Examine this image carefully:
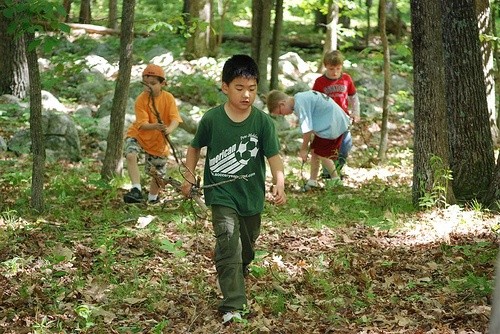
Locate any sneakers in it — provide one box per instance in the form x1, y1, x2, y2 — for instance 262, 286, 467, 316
222, 312, 241, 325
123, 187, 145, 203
147, 196, 160, 204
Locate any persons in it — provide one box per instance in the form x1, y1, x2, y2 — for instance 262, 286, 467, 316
123, 65, 182, 204
267, 90, 352, 191
182, 54, 286, 326
312, 50, 361, 180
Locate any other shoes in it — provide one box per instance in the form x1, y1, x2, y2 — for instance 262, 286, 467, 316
305, 179, 327, 192
331, 177, 341, 184
320, 173, 342, 180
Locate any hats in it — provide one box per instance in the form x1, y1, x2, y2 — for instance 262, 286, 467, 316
142, 65, 166, 80
